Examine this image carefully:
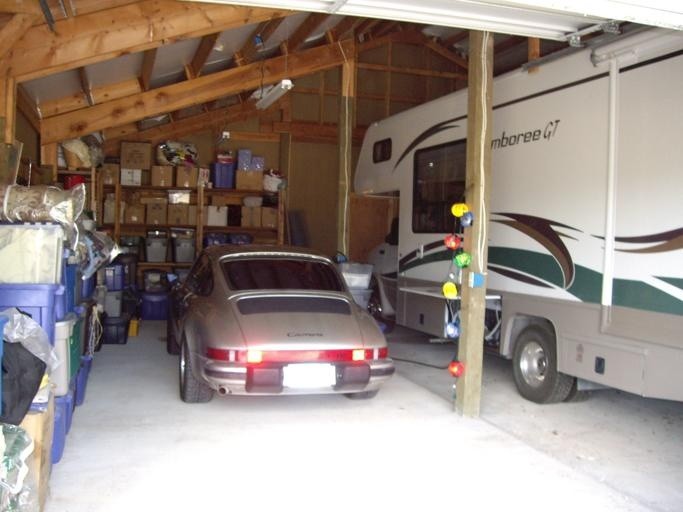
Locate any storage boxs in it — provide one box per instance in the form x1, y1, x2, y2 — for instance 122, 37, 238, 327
0, 249, 93, 511
94, 140, 277, 345
342, 262, 374, 308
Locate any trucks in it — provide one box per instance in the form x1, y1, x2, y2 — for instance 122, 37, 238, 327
352, 25, 683, 405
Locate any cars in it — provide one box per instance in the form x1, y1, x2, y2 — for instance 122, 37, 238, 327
167, 245, 395, 404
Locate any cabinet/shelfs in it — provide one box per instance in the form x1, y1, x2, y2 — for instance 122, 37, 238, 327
58, 164, 285, 269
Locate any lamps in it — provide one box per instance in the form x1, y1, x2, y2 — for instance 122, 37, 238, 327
255, 18, 293, 112
